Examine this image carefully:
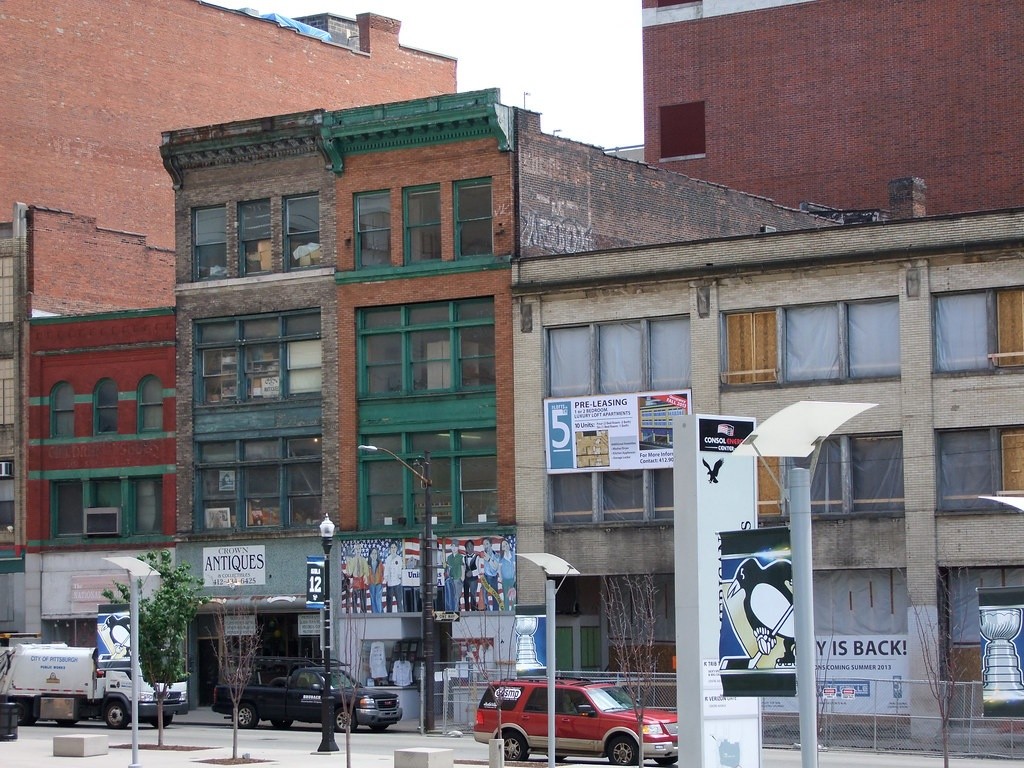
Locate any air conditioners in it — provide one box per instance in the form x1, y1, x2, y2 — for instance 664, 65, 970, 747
0, 462, 14, 478
82, 506, 123, 536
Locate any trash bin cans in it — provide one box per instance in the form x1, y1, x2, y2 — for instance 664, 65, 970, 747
0, 703, 22, 739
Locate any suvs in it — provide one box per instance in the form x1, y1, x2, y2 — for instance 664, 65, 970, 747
473, 676, 681, 766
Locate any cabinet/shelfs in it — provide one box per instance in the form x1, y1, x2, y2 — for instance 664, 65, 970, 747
221, 349, 279, 400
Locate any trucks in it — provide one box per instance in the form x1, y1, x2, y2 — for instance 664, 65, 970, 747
1, 639, 189, 728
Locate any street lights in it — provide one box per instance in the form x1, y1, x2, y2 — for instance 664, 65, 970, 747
356, 443, 450, 734
317, 512, 347, 752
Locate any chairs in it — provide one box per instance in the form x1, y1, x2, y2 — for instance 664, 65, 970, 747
560, 694, 577, 714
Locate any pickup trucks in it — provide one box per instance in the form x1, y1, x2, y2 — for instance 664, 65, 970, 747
211, 667, 403, 732
